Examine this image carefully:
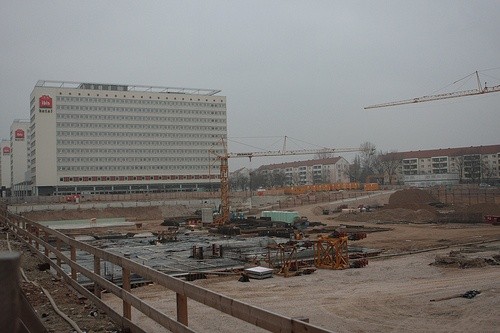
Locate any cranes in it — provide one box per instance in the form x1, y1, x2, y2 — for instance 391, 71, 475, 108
209, 135, 377, 227
364, 65, 500, 110
